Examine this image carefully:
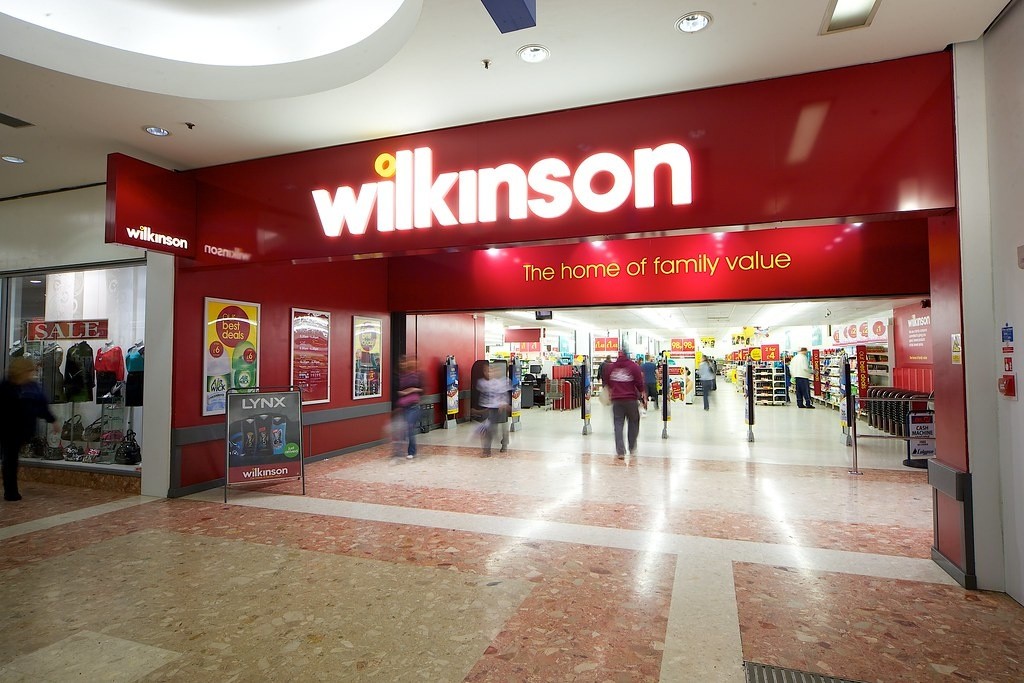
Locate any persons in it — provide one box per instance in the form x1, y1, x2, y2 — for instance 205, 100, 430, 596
597, 353, 659, 409
478, 363, 520, 458
10, 340, 24, 358
0, 358, 60, 501
398, 356, 423, 459
602, 349, 647, 460
699, 356, 717, 410
125, 341, 144, 406
790, 348, 816, 408
785, 358, 791, 403
64, 341, 96, 402
36, 341, 64, 398
94, 341, 124, 404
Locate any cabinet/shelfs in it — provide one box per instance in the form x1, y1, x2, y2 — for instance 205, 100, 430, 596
590, 355, 619, 397
722, 346, 890, 409
